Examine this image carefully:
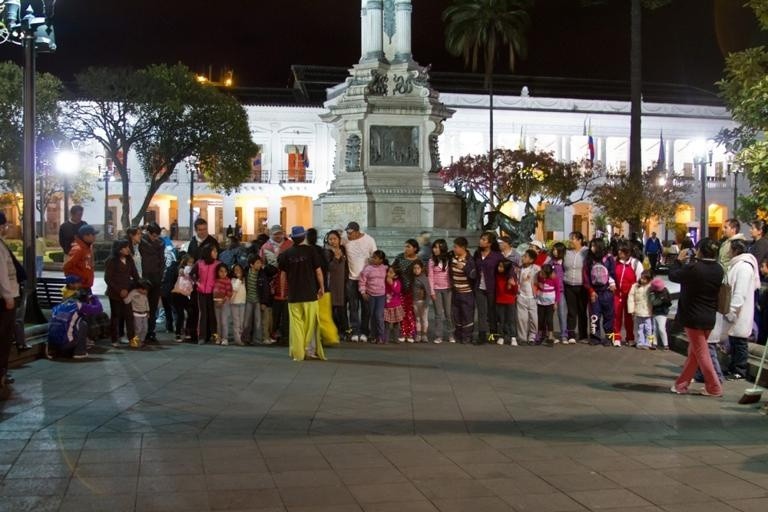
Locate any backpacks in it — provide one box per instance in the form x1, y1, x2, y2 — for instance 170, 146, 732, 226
590, 252, 611, 285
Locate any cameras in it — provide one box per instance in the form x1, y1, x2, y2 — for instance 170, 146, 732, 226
688, 249, 696, 256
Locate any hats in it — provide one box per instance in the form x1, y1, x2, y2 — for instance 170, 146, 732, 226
344, 222, 359, 231
269, 225, 283, 235
78, 224, 100, 237
63, 275, 82, 283
288, 226, 309, 238
651, 279, 665, 292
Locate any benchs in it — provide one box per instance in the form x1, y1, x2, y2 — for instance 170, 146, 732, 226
32, 277, 67, 309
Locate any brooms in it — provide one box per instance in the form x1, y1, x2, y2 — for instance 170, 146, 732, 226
738, 338, 768, 404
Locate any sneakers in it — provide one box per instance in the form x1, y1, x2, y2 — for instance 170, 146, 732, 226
726, 372, 746, 382
670, 384, 680, 394
44, 342, 53, 360
120, 339, 129, 343
86, 336, 95, 350
73, 352, 89, 359
111, 342, 120, 347
173, 329, 282, 345
699, 386, 721, 396
351, 334, 669, 350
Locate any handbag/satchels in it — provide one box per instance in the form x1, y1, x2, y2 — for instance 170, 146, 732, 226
717, 283, 732, 313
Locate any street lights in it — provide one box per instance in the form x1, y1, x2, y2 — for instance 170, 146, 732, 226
56, 149, 82, 222
726, 152, 744, 221
187, 152, 200, 241
192, 64, 237, 92
95, 154, 117, 240
691, 136, 719, 240
0, 0, 58, 349
658, 175, 669, 247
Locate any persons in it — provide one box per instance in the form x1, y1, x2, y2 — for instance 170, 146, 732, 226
665, 239, 725, 400
720, 237, 762, 381
690, 311, 725, 385
10, 317, 33, 354
1, 209, 29, 385
44, 204, 768, 363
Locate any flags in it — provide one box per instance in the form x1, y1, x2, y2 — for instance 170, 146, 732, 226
585, 126, 595, 172
658, 133, 666, 170
